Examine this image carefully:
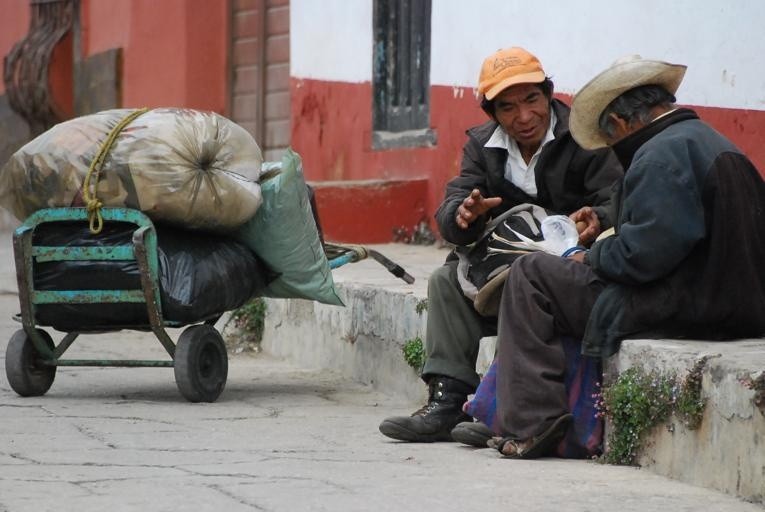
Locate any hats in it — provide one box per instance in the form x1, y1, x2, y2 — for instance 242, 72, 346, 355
566, 54, 690, 155
476, 47, 548, 103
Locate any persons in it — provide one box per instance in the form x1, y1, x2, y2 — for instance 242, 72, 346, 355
488, 54, 764, 460
380, 47, 624, 447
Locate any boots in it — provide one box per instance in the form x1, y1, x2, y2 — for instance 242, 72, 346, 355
448, 416, 496, 449
376, 376, 472, 442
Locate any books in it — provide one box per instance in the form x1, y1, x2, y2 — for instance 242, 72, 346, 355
489, 213, 615, 259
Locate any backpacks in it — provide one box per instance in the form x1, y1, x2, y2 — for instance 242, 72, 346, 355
457, 203, 560, 318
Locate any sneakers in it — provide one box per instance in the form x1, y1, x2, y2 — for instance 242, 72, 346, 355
486, 410, 574, 460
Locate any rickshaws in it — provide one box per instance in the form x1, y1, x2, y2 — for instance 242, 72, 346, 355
4, 205, 416, 402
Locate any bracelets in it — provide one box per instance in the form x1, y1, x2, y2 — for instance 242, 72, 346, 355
561, 246, 586, 256
